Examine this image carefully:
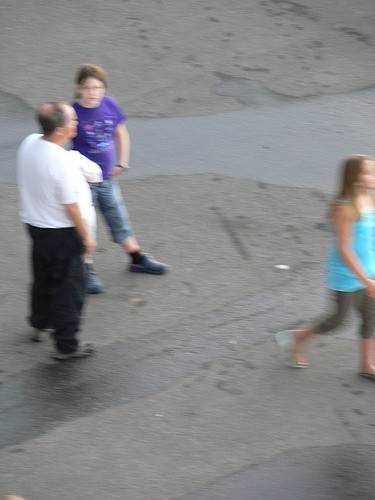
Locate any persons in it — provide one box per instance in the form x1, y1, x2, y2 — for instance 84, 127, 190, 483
16, 102, 98, 360
275, 155, 375, 379
64, 63, 169, 294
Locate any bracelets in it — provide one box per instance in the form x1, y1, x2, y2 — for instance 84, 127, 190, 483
118, 164, 130, 172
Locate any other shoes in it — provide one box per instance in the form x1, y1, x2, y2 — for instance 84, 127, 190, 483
52, 342, 94, 358
31, 329, 53, 341
83, 270, 105, 292
129, 255, 165, 273
360, 369, 375, 379
